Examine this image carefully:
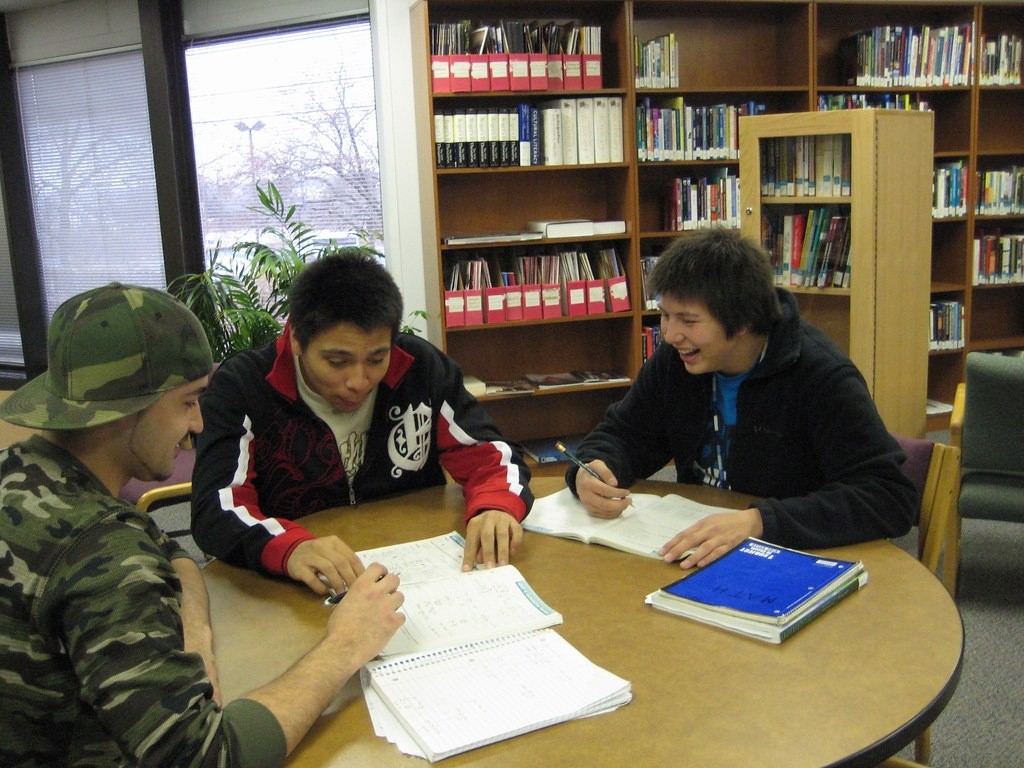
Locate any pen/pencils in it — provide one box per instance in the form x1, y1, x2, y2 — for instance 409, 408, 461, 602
554, 441, 637, 510
324, 568, 402, 606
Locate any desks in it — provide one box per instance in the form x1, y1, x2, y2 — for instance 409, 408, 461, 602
194, 475, 966, 768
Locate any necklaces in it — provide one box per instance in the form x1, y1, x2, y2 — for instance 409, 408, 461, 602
713, 339, 768, 489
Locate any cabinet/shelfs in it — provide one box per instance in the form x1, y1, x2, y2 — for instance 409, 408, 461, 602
410, 0, 1024, 476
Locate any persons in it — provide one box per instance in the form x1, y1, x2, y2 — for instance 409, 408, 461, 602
0, 280, 406, 768
189, 255, 534, 594
564, 226, 920, 569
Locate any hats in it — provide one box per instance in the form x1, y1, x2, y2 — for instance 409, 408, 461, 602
0, 281, 213, 429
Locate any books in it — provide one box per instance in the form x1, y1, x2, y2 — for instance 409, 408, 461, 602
651, 536, 868, 642
520, 484, 738, 561
317, 530, 631, 763
430, 18, 1024, 464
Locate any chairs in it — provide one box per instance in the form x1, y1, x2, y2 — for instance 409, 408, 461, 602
118, 363, 222, 561
874, 435, 961, 768
943, 354, 1024, 600
